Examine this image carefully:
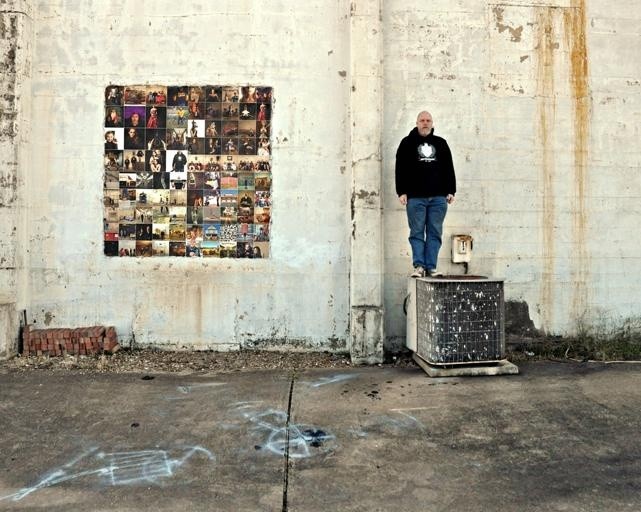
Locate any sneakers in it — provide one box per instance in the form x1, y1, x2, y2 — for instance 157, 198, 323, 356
427, 268, 443, 278
410, 265, 426, 277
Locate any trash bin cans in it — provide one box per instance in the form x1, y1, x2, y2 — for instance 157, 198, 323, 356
402, 274, 506, 366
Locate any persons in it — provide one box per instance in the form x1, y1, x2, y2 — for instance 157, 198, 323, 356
104, 84, 272, 258
393, 109, 458, 279
